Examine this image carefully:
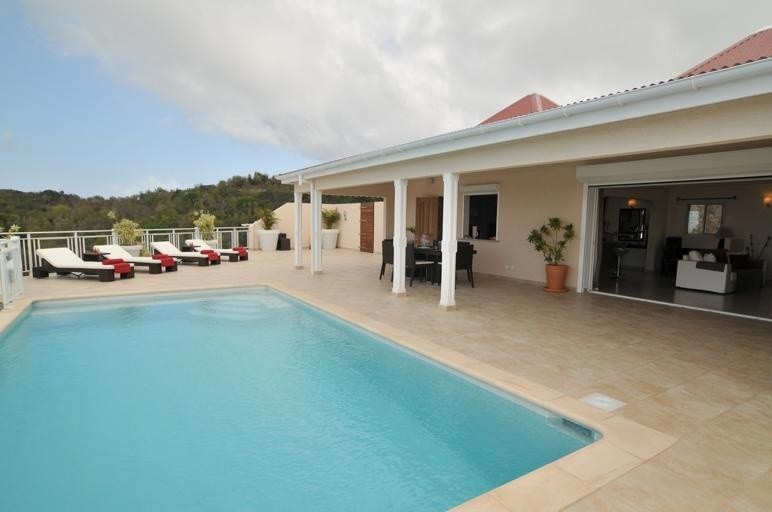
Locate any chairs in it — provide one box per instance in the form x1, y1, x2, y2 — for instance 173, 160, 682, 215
32, 238, 256, 283
371, 238, 480, 289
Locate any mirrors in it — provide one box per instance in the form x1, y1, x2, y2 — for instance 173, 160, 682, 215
617, 207, 644, 242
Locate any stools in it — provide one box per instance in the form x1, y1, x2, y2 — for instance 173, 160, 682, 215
612, 246, 629, 280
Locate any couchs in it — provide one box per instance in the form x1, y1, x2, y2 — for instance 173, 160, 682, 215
673, 255, 737, 294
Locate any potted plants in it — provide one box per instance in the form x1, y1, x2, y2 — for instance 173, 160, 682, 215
194, 209, 220, 255
528, 210, 573, 295
318, 206, 342, 250
114, 215, 146, 257
256, 203, 286, 255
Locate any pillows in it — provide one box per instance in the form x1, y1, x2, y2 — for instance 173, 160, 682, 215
703, 252, 716, 262
688, 250, 703, 261
